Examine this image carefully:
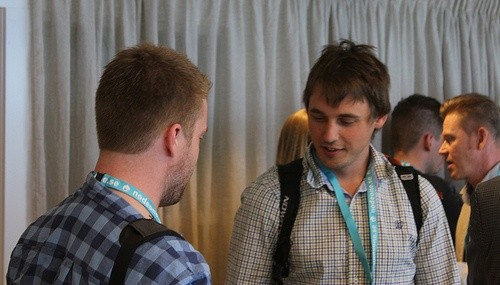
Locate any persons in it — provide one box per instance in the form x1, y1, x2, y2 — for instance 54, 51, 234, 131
381, 94, 464, 268
275, 108, 314, 166
1, 41, 212, 285
440, 92, 500, 284
221, 39, 463, 284
